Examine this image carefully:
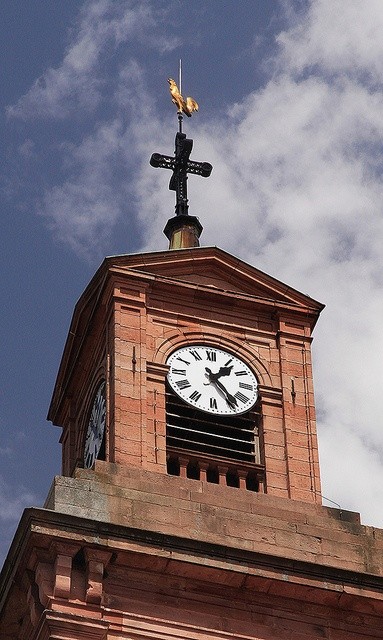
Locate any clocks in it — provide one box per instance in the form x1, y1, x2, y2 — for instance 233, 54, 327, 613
81, 380, 106, 468
165, 341, 261, 419
